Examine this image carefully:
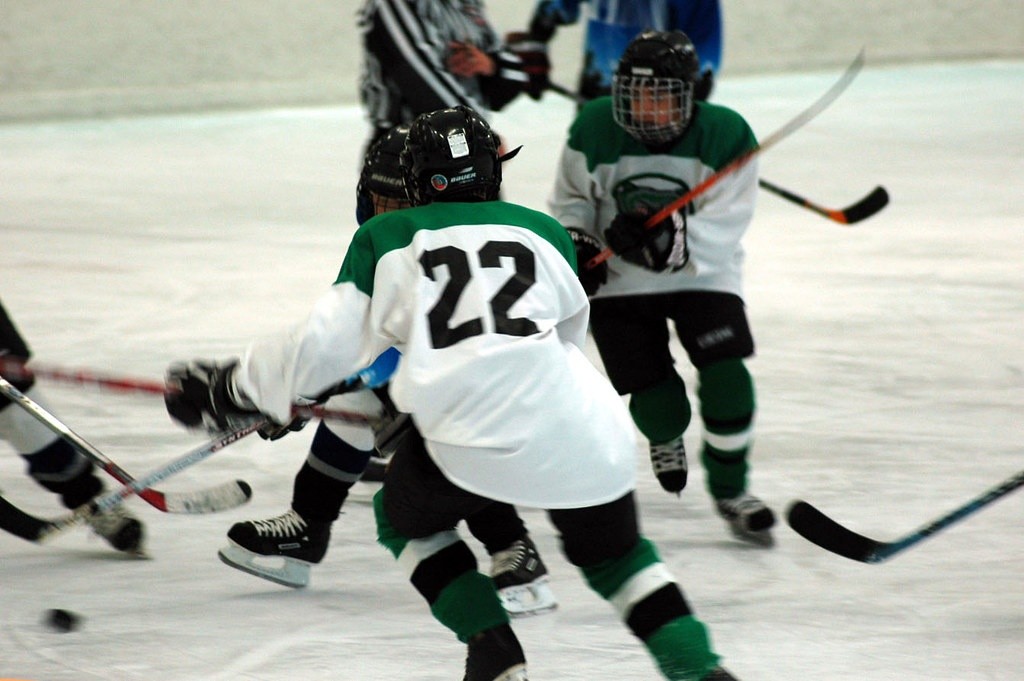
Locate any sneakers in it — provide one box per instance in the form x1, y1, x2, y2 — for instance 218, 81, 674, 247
718, 493, 775, 547
218, 507, 328, 586
488, 534, 557, 614
650, 435, 688, 497
87, 502, 147, 558
463, 625, 528, 681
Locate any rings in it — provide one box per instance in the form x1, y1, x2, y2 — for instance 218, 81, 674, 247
464, 49, 477, 65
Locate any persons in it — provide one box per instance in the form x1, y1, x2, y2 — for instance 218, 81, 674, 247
165, 105, 738, 681
226, 126, 548, 592
356, 0, 724, 158
549, 32, 775, 532
0, 302, 142, 552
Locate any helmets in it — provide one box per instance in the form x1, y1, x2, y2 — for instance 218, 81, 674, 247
611, 28, 696, 141
400, 105, 502, 206
355, 124, 411, 218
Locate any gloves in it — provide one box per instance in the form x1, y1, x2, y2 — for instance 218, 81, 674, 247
509, 30, 549, 99
602, 204, 688, 273
163, 360, 259, 427
566, 228, 608, 296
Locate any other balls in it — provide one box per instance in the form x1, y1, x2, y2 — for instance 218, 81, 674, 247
41, 609, 75, 631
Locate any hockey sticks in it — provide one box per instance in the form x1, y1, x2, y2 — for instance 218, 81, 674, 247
787, 470, 1024, 566
583, 49, 866, 268
0, 376, 253, 513
543, 77, 890, 225
0, 418, 269, 545
0, 360, 379, 427
